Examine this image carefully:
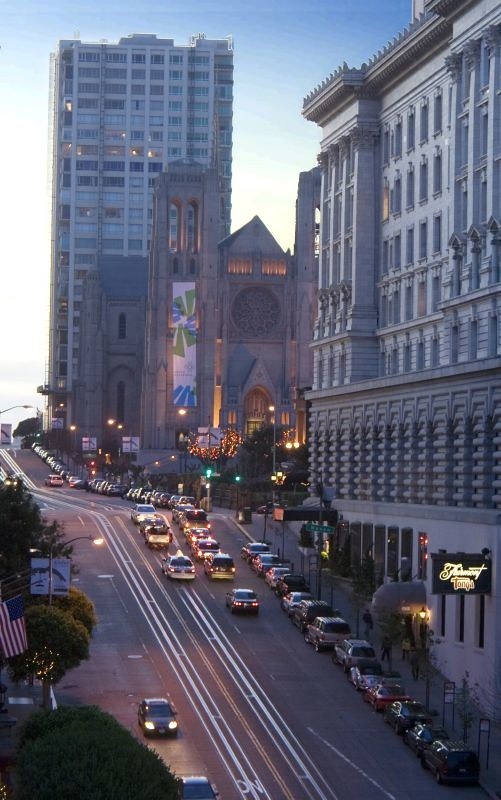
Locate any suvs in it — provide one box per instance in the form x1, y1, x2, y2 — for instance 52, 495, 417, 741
288, 599, 378, 674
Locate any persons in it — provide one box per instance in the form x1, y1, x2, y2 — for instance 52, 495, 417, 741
380, 635, 390, 661
362, 609, 374, 636
401, 635, 420, 681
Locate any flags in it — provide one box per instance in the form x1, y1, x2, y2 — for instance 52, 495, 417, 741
0, 594, 29, 658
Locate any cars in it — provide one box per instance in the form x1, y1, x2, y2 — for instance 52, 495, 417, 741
137, 698, 179, 737
160, 553, 196, 580
44, 475, 63, 487
281, 591, 314, 615
258, 502, 287, 514
226, 589, 259, 615
348, 663, 449, 755
187, 528, 236, 582
177, 777, 218, 800
131, 504, 173, 550
32, 442, 212, 536
241, 543, 292, 590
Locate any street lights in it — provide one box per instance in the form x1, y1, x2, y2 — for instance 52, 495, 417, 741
177, 407, 210, 512
268, 405, 277, 507
70, 424, 91, 480
43, 535, 103, 705
419, 607, 428, 648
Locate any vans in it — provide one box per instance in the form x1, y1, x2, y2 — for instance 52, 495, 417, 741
428, 739, 480, 786
